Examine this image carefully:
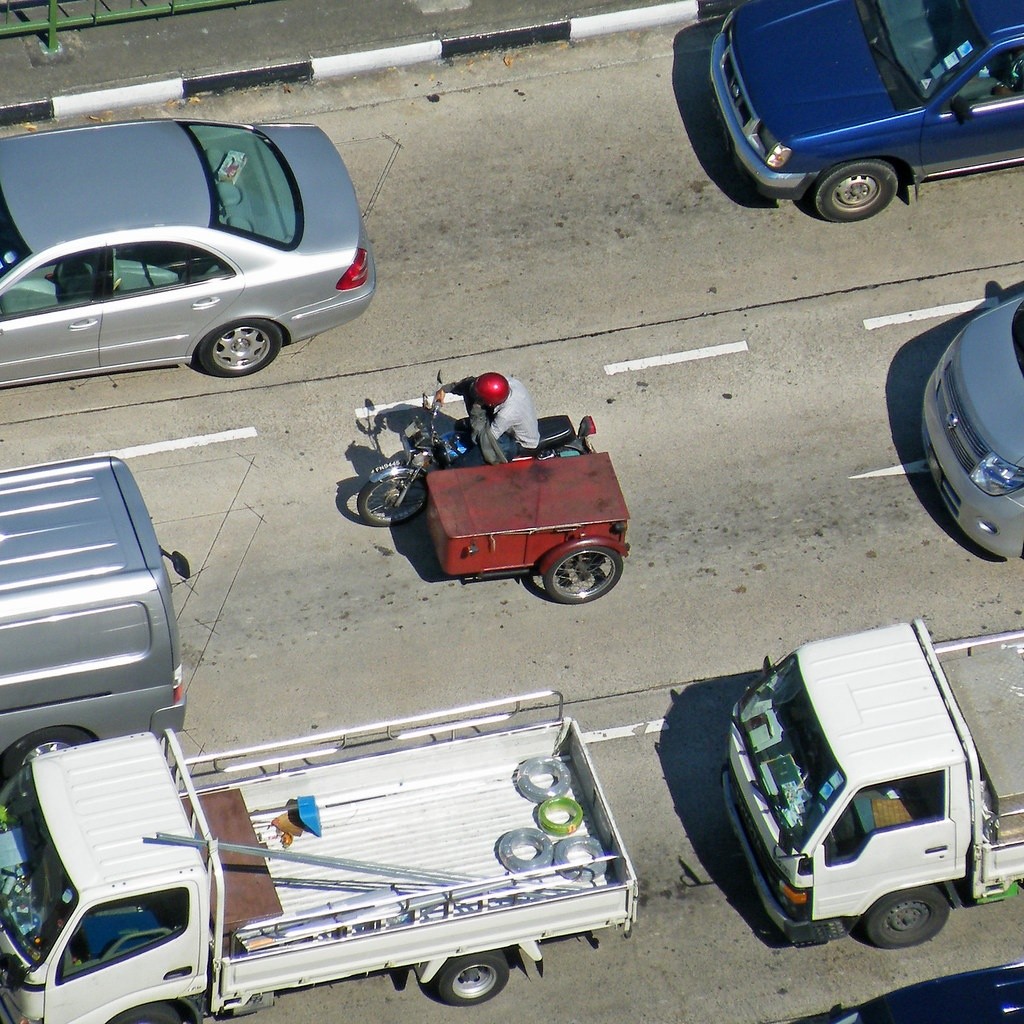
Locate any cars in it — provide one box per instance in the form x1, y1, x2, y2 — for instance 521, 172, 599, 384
782, 958, 1024, 1024
920, 290, 1024, 559
1, 116, 376, 378
708, 0, 1024, 223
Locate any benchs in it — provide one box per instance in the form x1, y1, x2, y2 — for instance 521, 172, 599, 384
204, 145, 258, 236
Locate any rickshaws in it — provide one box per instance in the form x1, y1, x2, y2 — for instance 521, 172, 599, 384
356, 367, 632, 606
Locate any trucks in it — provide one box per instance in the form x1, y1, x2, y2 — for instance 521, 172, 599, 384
722, 616, 1023, 951
0, 682, 639, 1024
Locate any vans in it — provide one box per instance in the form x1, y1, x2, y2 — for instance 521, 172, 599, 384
0, 454, 191, 785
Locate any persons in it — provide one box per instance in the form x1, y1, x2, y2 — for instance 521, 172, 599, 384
433, 372, 541, 467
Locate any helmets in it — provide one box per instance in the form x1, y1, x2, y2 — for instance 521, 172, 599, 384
475, 372, 509, 407
